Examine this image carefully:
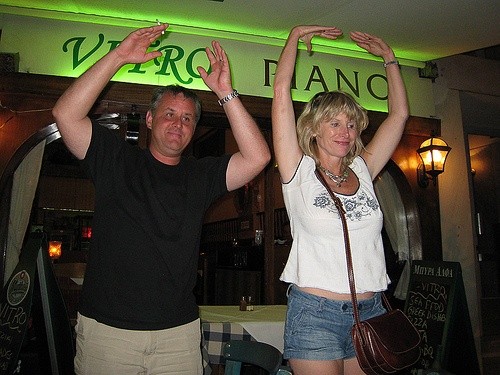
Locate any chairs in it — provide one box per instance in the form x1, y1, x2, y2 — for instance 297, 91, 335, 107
220, 341, 285, 375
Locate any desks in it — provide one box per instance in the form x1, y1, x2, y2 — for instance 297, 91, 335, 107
199, 305, 287, 375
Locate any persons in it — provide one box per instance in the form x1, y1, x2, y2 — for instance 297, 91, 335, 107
272, 26, 410, 375
52, 22, 272, 375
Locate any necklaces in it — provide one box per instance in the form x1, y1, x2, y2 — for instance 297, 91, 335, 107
320, 165, 348, 186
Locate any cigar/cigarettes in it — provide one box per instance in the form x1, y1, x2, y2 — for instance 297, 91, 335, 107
157, 22, 164, 34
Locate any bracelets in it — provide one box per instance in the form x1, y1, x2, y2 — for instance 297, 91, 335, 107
383, 60, 399, 67
218, 89, 239, 106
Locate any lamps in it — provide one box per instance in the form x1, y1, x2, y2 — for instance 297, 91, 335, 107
416, 129, 452, 189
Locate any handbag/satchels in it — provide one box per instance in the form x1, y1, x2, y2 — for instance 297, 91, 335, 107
351, 309, 423, 375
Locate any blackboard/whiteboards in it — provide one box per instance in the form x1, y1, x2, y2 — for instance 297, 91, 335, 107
405, 259, 481, 374
